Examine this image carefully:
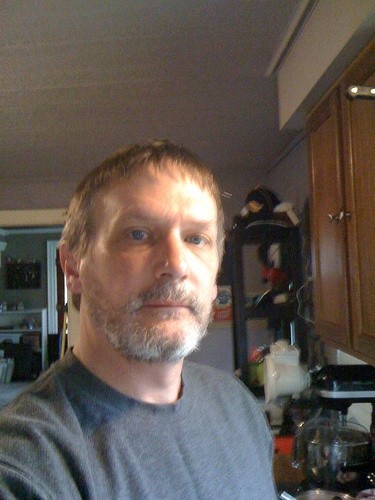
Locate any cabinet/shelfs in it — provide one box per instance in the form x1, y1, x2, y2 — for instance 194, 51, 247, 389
305, 39, 375, 362
0, 308, 49, 380
229, 213, 306, 397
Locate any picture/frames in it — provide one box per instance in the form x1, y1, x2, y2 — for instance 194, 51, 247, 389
7, 261, 41, 290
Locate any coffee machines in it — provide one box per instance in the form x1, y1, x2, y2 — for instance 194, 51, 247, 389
292, 363, 375, 496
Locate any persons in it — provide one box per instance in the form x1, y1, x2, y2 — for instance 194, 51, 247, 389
0, 138, 277, 500
